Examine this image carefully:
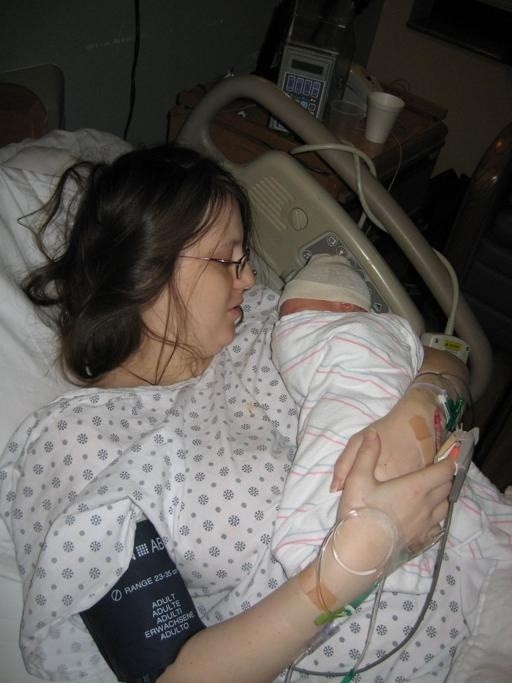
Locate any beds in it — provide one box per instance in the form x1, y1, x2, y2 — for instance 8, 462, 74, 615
0, 64, 512, 683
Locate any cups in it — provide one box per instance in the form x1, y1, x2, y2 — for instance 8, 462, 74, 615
366, 92, 404, 143
329, 101, 363, 144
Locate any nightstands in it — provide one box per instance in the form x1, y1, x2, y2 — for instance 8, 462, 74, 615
167, 69, 448, 241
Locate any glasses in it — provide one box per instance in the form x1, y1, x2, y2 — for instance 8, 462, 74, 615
177, 249, 252, 280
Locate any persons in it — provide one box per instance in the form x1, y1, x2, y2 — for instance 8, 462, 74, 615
0, 141, 472, 683
280, 251, 372, 318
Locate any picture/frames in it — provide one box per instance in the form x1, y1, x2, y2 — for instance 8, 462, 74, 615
407, 0, 512, 64
